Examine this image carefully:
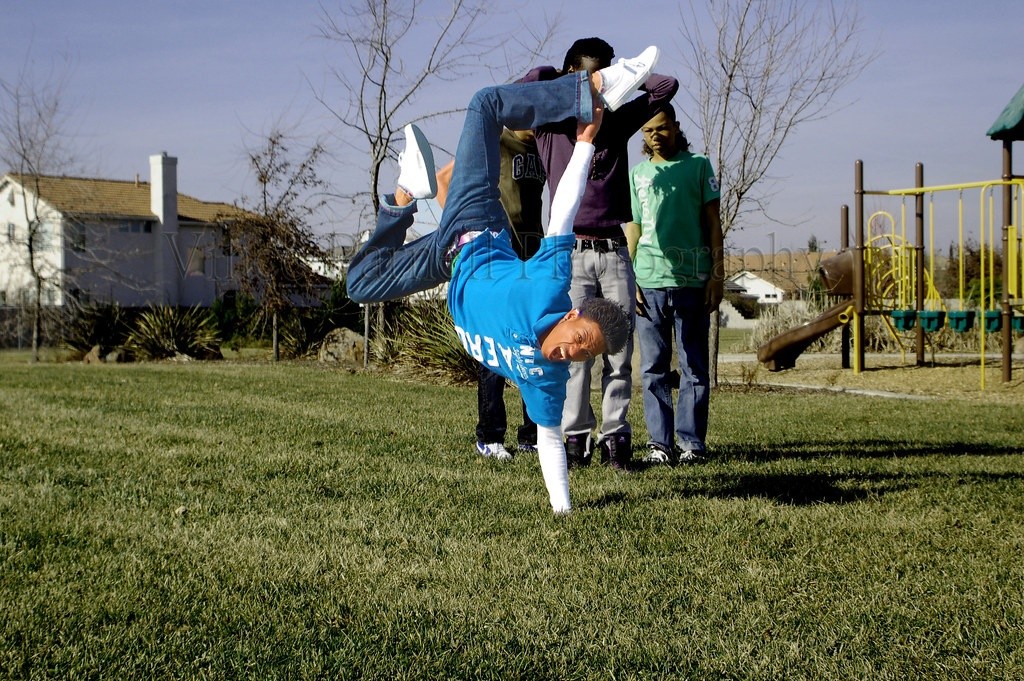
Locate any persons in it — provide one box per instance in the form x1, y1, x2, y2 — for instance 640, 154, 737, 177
345, 45, 660, 512
625, 102, 725, 463
436, 125, 547, 462
511, 37, 679, 472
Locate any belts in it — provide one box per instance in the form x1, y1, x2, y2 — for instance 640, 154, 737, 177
574, 237, 627, 253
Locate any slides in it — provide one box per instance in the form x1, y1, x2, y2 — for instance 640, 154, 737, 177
757, 298, 855, 370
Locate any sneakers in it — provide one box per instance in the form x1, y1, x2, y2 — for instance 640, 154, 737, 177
679, 452, 704, 462
518, 444, 538, 451
476, 440, 513, 460
564, 432, 595, 472
599, 46, 660, 112
641, 445, 675, 466
597, 432, 633, 470
398, 124, 437, 198
